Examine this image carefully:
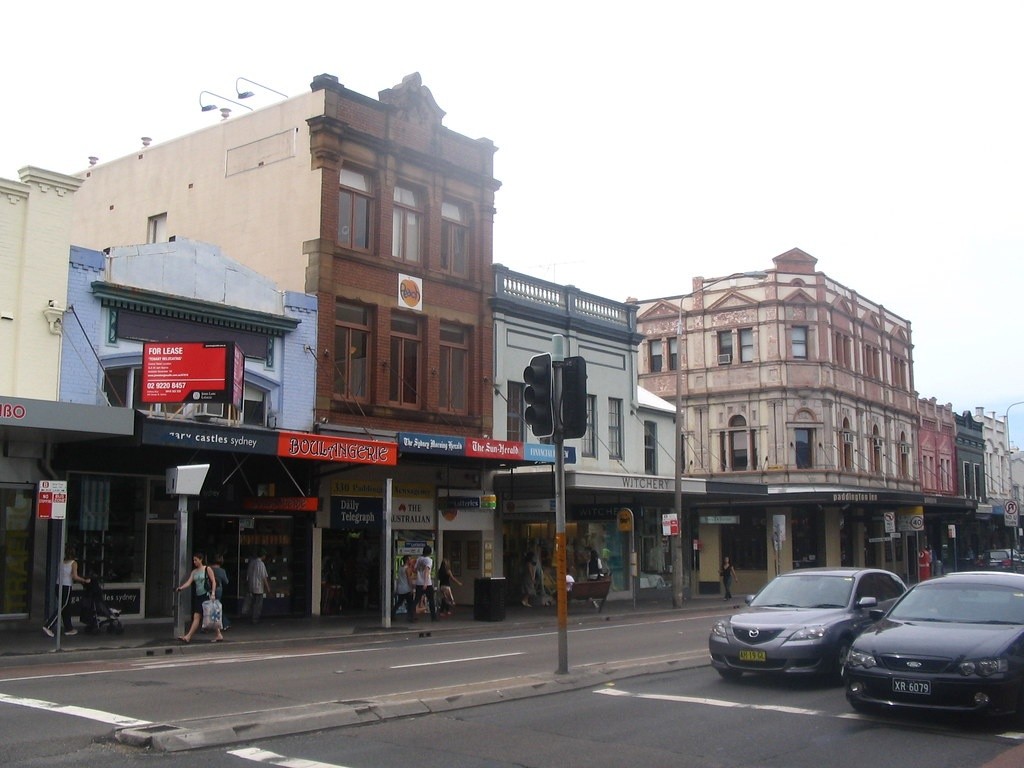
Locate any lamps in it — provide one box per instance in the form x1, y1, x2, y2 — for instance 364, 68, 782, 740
236, 77, 290, 99
313, 417, 329, 436
199, 90, 254, 113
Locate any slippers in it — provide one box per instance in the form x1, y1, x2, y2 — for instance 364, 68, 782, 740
209, 636, 224, 642
178, 635, 190, 644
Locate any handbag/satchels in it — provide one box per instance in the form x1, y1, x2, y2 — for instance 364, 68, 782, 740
204, 566, 217, 592
202, 598, 223, 630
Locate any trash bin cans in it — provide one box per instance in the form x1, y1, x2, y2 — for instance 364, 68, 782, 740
473, 576, 508, 622
934, 560, 943, 577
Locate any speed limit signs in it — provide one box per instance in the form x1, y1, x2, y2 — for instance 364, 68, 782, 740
910, 516, 923, 529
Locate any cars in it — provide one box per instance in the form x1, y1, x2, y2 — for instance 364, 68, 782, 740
982, 548, 1024, 566
708, 568, 908, 681
840, 572, 1024, 732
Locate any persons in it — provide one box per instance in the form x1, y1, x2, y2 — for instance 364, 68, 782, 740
246, 547, 270, 625
543, 567, 555, 596
437, 559, 464, 617
520, 551, 538, 608
406, 545, 440, 623
42, 546, 91, 638
391, 555, 420, 621
586, 549, 603, 609
718, 556, 738, 601
565, 568, 576, 616
175, 552, 224, 643
200, 553, 232, 633
919, 544, 946, 582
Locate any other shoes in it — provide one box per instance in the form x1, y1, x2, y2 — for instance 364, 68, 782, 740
42, 626, 55, 638
520, 599, 534, 608
64, 628, 79, 635
222, 625, 231, 632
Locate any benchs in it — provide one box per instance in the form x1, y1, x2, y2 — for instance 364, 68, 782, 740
551, 581, 612, 614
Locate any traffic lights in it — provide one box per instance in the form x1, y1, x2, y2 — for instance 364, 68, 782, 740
523, 353, 555, 439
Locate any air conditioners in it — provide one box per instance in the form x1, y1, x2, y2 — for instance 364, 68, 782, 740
194, 402, 224, 417
718, 354, 732, 364
843, 432, 910, 455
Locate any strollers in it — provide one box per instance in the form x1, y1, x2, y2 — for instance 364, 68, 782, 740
81, 575, 124, 635
